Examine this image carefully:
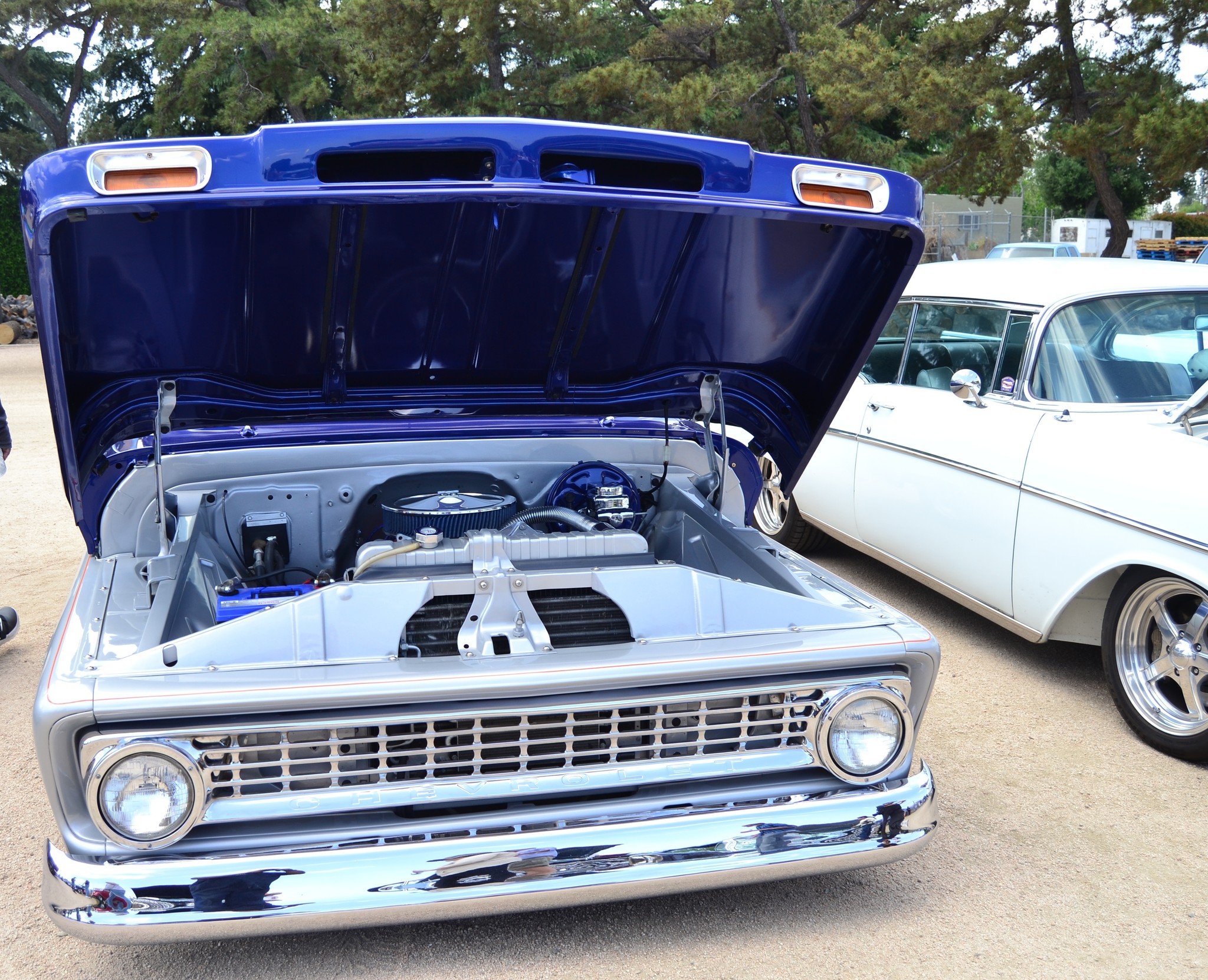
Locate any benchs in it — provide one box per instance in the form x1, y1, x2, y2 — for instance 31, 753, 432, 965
862, 338, 1070, 383
915, 350, 1191, 401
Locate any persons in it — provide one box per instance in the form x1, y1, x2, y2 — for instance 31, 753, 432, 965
0, 401, 19, 644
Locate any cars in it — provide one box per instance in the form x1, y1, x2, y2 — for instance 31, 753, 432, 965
985, 243, 1081, 259
1185, 244, 1208, 265
20, 116, 944, 944
698, 257, 1208, 769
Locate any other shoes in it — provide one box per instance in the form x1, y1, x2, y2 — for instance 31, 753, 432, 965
0, 607, 20, 646
